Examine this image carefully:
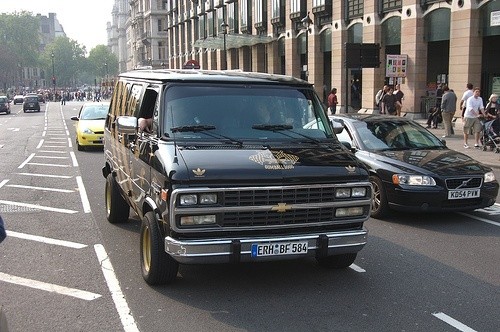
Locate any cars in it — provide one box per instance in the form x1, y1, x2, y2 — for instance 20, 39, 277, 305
0, 96, 12, 115
26, 93, 45, 103
302, 112, 500, 219
13, 94, 24, 105
22, 100, 41, 113
70, 102, 111, 151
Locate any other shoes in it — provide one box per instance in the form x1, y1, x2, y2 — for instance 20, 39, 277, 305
426, 121, 431, 125
474, 144, 482, 149
426, 125, 431, 128
442, 135, 445, 137
464, 144, 470, 148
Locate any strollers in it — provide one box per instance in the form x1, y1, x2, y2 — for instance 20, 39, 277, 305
477, 114, 500, 153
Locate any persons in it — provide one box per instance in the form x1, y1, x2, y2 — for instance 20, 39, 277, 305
426, 103, 443, 129
138, 118, 152, 134
375, 83, 404, 116
45, 89, 112, 105
442, 86, 457, 138
462, 87, 488, 149
478, 93, 500, 145
328, 88, 338, 115
253, 99, 293, 128
460, 82, 475, 110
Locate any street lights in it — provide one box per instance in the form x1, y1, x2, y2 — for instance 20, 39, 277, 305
220, 21, 229, 70
104, 63, 108, 93
51, 53, 56, 94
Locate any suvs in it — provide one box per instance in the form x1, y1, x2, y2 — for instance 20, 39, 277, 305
102, 67, 375, 286
